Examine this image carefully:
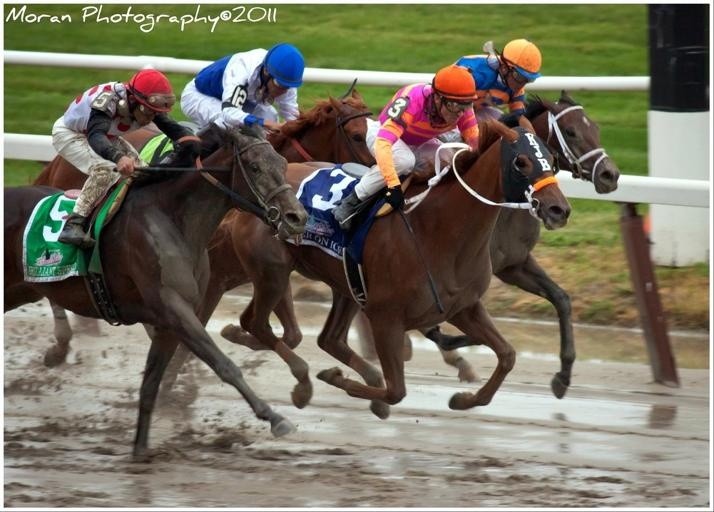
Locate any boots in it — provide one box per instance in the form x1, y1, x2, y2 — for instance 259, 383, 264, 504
330, 187, 364, 231
57, 212, 96, 248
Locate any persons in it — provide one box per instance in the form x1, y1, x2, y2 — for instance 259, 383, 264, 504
179, 44, 306, 143
51, 66, 195, 250
441, 39, 542, 134
333, 63, 481, 237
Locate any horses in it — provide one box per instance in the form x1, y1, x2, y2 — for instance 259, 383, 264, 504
33, 88, 391, 391
358, 90, 619, 401
3, 116, 307, 463
210, 113, 574, 419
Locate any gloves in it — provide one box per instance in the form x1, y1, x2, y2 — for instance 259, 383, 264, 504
381, 185, 406, 212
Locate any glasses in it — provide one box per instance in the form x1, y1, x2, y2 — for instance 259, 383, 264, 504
437, 95, 473, 114
135, 105, 163, 117
508, 67, 530, 84
270, 77, 288, 90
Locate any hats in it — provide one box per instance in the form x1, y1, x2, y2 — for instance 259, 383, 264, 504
123, 65, 176, 109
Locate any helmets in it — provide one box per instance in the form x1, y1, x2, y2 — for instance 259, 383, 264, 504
262, 42, 305, 87
500, 38, 543, 83
432, 64, 478, 102
